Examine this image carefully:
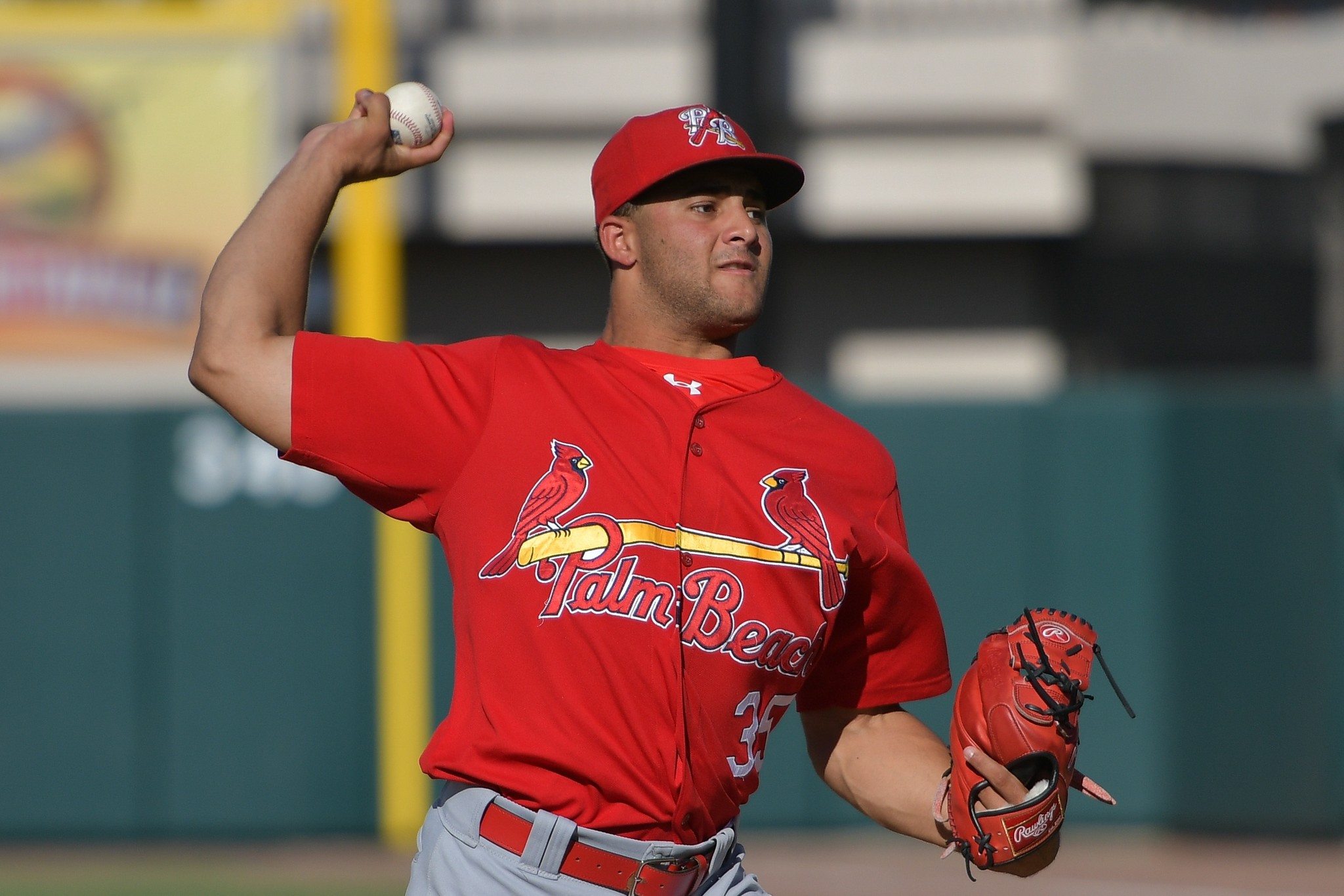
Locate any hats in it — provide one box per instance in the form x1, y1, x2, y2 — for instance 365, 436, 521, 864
592, 104, 805, 227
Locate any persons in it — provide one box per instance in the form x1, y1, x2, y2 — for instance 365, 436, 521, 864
188, 89, 1053, 896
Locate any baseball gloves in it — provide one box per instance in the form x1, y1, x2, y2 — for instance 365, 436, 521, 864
947, 607, 1097, 871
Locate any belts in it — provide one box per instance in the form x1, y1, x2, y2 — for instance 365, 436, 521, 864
480, 804, 712, 896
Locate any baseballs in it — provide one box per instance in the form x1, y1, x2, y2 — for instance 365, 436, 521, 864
383, 81, 445, 148
